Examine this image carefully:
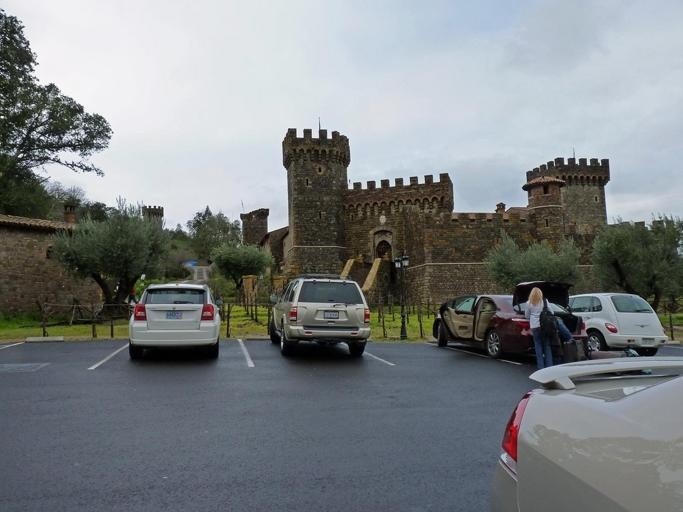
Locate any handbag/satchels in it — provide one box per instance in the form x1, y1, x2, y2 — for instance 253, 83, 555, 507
539, 309, 559, 345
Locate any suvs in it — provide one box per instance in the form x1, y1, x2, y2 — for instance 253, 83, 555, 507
267, 274, 371, 357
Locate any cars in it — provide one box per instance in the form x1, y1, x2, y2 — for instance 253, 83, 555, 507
490, 354, 681, 512
127, 281, 220, 359
433, 280, 587, 358
567, 293, 667, 355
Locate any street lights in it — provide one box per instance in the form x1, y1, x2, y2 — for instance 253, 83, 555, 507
393, 255, 410, 339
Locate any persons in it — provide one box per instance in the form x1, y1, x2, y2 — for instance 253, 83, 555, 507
127, 288, 139, 306
524, 287, 554, 370
